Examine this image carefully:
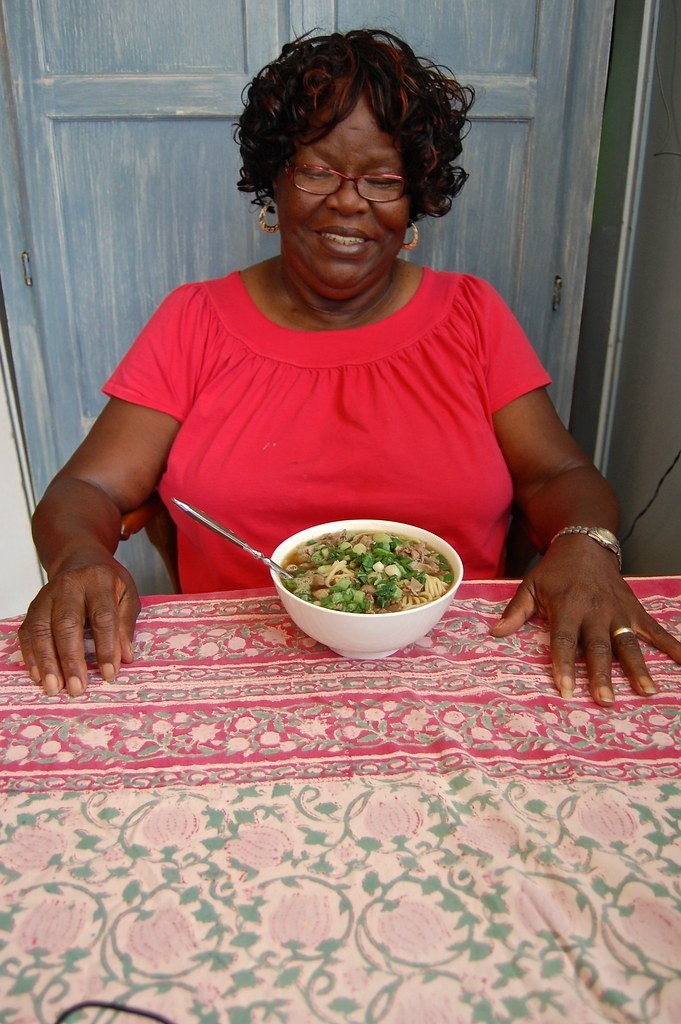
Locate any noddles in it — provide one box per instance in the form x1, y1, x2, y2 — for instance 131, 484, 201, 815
278, 528, 454, 615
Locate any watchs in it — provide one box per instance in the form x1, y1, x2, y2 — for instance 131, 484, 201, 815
550, 525, 622, 572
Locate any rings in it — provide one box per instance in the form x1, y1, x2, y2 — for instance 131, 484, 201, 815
612, 627, 634, 639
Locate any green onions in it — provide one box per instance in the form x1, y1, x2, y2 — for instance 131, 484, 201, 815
280, 532, 453, 614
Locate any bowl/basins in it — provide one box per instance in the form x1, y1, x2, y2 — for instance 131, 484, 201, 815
269, 517, 466, 664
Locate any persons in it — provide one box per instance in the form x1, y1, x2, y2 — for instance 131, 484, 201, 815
19, 25, 681, 706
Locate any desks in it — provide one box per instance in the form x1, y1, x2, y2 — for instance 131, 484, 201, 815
1, 574, 681, 1024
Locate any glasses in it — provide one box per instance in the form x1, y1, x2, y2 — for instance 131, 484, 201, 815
283, 161, 413, 203
